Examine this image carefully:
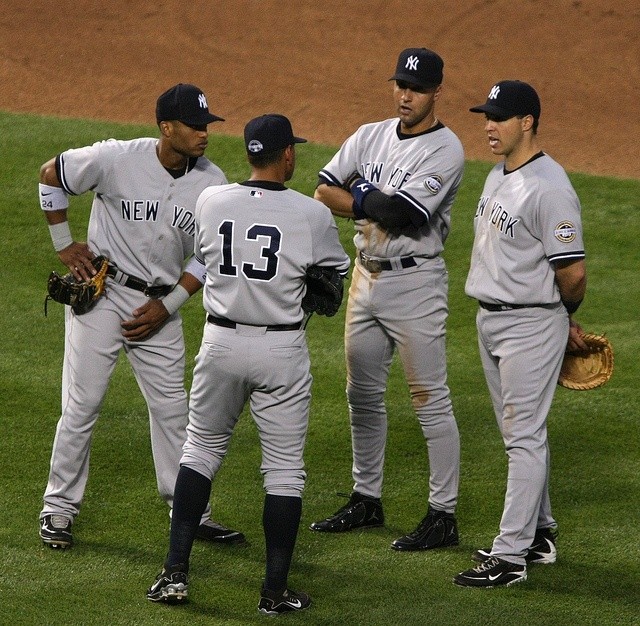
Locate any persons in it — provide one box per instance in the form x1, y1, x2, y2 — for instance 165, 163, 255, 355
312, 48, 466, 553
454, 80, 589, 589
38, 84, 247, 550
145, 113, 351, 615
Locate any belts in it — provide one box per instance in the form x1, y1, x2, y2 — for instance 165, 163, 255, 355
477, 299, 548, 312
206, 315, 302, 332
359, 252, 416, 274
104, 265, 172, 297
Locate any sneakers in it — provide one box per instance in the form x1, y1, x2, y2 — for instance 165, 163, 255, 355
391, 505, 460, 551
308, 492, 385, 533
452, 554, 527, 588
257, 585, 313, 615
145, 571, 188, 602
169, 516, 247, 543
469, 534, 557, 565
39, 514, 73, 548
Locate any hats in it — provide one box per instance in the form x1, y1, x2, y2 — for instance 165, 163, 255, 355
469, 79, 541, 117
243, 113, 307, 154
155, 84, 225, 126
387, 48, 444, 89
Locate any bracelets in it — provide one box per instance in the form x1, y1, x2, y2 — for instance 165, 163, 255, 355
350, 178, 380, 210
45, 220, 75, 252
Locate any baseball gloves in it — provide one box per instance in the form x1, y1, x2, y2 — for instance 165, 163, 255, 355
47, 255, 108, 313
302, 264, 344, 318
557, 333, 614, 391
